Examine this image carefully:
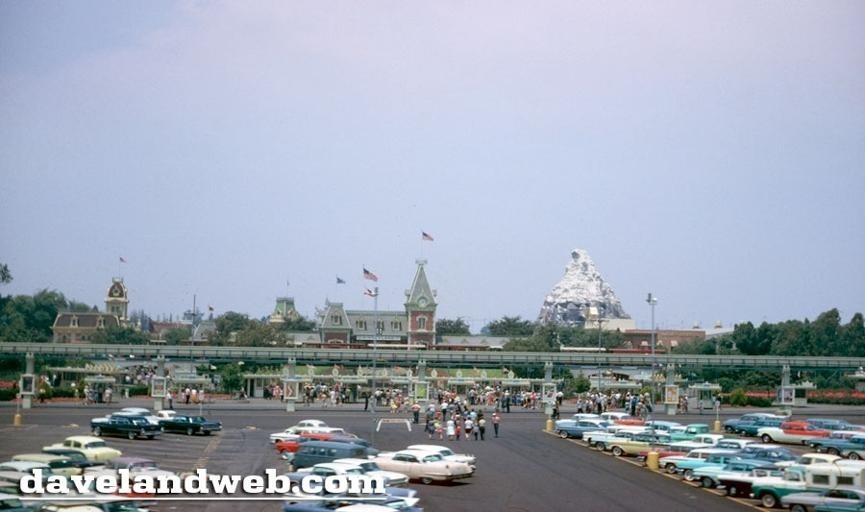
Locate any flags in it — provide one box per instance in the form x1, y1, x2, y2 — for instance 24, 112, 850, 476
364, 287, 380, 298
119, 255, 128, 264
422, 232, 435, 241
364, 268, 378, 281
337, 277, 346, 284
208, 306, 214, 312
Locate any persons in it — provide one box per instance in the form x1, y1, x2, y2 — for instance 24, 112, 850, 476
259, 380, 724, 443
239, 385, 247, 400
180, 389, 186, 407
80, 363, 157, 407
167, 390, 175, 411
191, 386, 198, 404
185, 386, 191, 405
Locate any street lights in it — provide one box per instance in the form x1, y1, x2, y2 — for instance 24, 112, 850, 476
647, 291, 658, 470
586, 317, 610, 391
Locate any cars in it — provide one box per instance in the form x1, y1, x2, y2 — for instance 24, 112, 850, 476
1, 434, 159, 512
269, 418, 476, 512
91, 406, 223, 441
